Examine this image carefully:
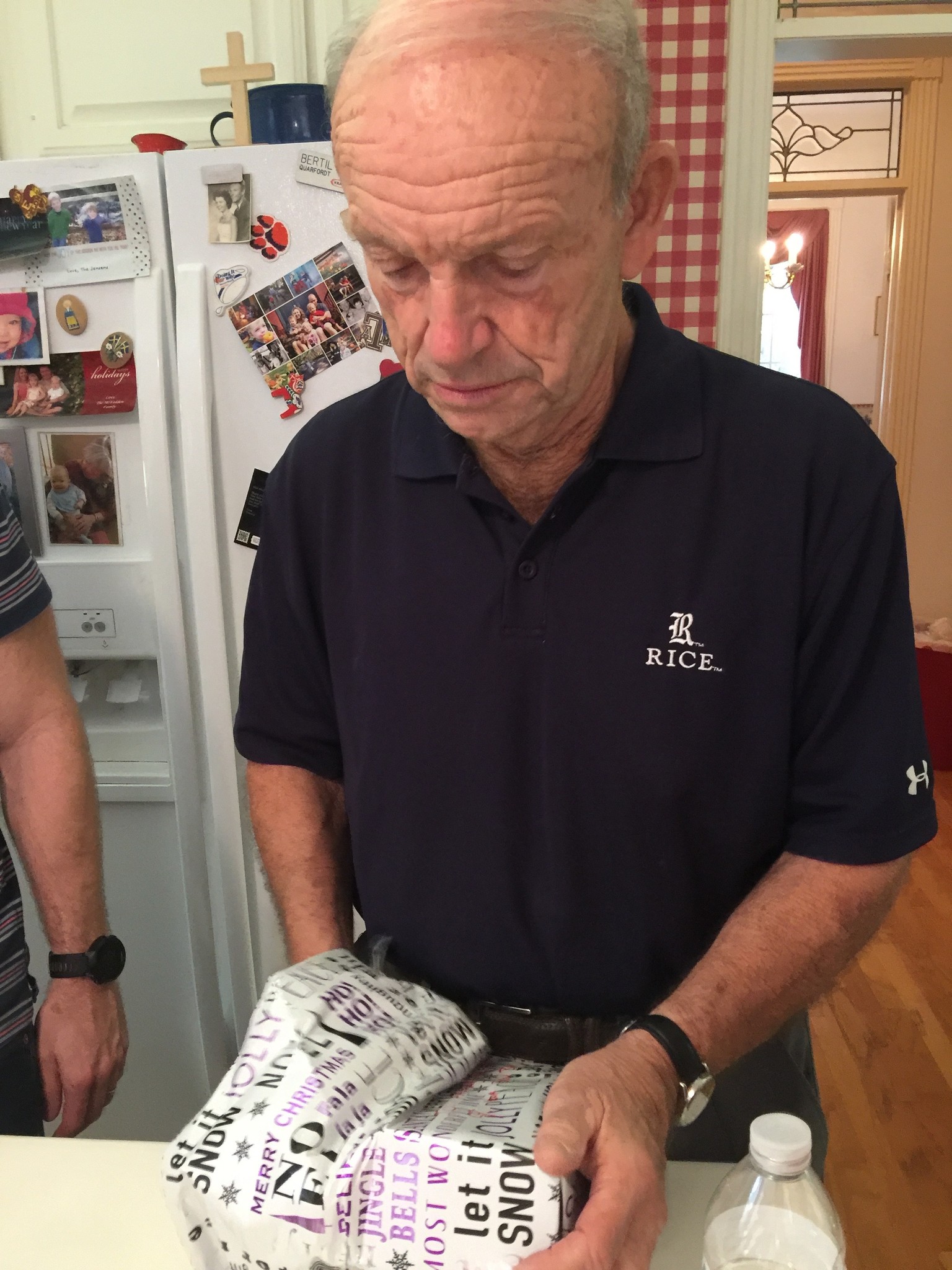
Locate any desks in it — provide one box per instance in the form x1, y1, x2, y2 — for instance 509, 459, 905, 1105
1, 1135, 742, 1270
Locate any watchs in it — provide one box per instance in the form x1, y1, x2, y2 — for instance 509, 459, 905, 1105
48, 936, 126, 985
617, 1012, 716, 1131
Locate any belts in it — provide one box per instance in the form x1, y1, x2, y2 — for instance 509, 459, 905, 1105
350, 930, 637, 1067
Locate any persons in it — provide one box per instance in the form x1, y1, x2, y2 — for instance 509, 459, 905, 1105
80, 203, 111, 242
212, 190, 238, 243
0, 495, 128, 1139
6, 365, 70, 417
0, 293, 41, 359
229, 179, 250, 241
226, 243, 374, 394
47, 464, 92, 545
235, 3, 938, 1270
46, 193, 74, 247
44, 443, 117, 545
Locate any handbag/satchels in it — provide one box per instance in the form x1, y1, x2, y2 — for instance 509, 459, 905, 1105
165, 948, 587, 1270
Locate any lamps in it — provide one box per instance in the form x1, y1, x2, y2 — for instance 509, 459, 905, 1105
763, 231, 805, 291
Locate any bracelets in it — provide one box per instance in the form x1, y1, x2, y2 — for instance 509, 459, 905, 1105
92, 513, 99, 524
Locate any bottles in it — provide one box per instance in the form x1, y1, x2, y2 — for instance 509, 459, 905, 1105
700, 1112, 846, 1270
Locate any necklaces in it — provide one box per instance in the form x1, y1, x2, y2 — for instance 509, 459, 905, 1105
12, 346, 17, 359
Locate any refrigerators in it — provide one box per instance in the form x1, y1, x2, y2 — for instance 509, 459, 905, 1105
0, 143, 411, 1141
